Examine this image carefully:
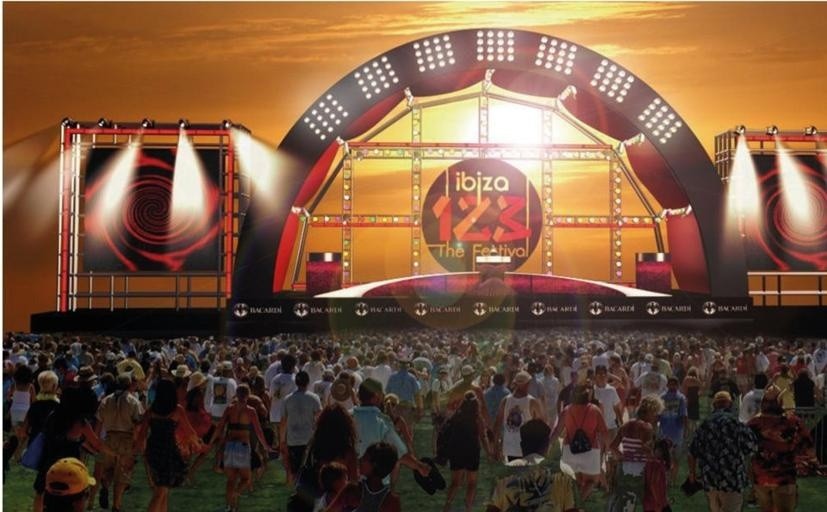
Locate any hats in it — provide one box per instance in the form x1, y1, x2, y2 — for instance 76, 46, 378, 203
46, 457, 96, 496
461, 365, 474, 377
74, 366, 98, 383
516, 371, 531, 384
332, 378, 352, 400
714, 391, 732, 402
171, 364, 207, 391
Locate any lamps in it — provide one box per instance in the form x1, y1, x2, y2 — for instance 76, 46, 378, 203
62, 116, 234, 130
735, 123, 820, 138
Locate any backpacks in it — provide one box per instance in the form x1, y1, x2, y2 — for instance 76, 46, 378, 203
571, 404, 592, 453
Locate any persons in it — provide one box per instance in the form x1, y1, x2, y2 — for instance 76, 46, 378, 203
4, 326, 826, 512
